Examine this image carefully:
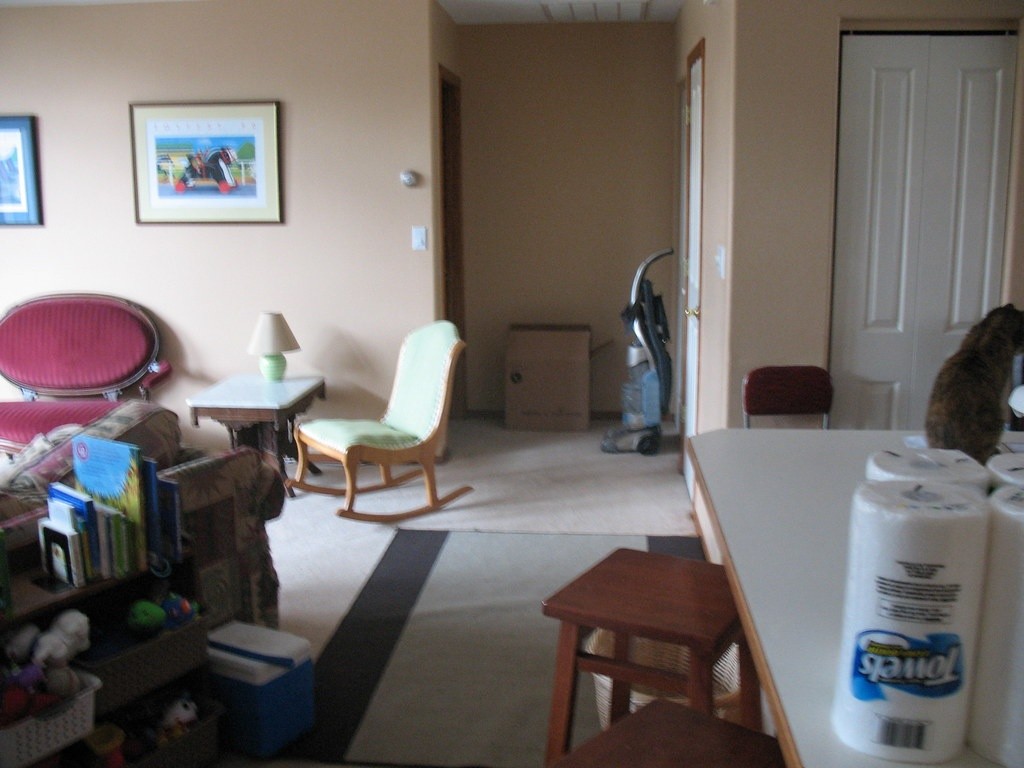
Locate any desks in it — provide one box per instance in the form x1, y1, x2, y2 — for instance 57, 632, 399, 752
187, 375, 326, 499
684, 427, 1024, 768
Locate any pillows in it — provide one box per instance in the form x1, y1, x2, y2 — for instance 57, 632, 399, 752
12, 396, 183, 502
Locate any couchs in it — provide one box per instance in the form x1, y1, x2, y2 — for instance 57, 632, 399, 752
0, 444, 286, 630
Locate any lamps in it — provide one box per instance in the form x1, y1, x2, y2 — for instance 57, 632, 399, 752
247, 311, 300, 384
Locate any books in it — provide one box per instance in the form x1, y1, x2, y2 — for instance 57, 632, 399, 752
38, 435, 180, 588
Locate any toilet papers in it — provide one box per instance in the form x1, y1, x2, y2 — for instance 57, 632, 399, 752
829, 441, 1023, 767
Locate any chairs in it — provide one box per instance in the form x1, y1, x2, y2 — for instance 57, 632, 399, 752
0, 294, 173, 466
741, 364, 834, 430
282, 320, 476, 524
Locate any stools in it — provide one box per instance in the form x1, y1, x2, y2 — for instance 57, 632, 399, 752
541, 547, 742, 768
552, 696, 785, 768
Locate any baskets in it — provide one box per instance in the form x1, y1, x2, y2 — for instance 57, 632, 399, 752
129, 692, 229, 768
69, 596, 212, 722
0, 667, 103, 768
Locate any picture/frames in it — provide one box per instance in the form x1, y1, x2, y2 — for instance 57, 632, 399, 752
129, 100, 284, 225
0, 115, 43, 226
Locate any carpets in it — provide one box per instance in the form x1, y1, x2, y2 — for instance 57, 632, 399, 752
265, 524, 705, 768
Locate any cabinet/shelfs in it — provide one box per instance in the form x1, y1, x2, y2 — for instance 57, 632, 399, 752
0, 530, 206, 635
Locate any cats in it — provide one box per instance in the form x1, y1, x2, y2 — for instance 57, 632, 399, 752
925, 303, 1024, 466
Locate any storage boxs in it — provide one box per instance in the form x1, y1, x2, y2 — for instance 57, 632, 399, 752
0, 669, 102, 768
502, 323, 615, 432
73, 687, 225, 768
78, 614, 212, 716
206, 619, 318, 760
